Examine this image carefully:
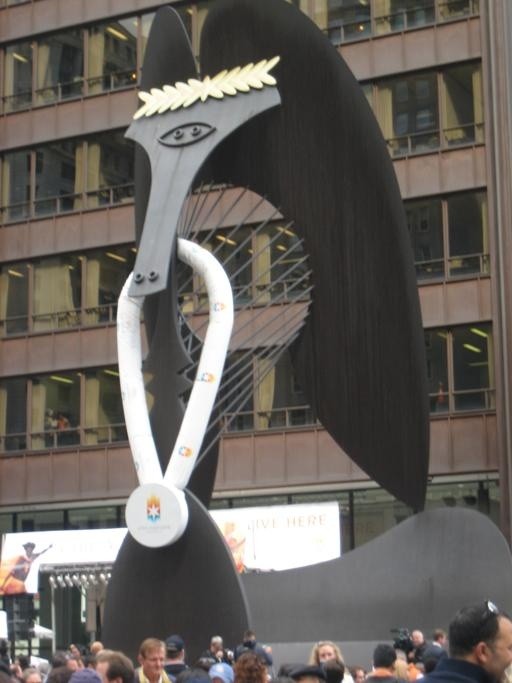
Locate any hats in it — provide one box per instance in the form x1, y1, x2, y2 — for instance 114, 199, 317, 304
164, 634, 185, 653
67, 668, 103, 683
208, 662, 234, 683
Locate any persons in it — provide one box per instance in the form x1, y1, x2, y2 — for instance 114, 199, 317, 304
45, 408, 72, 437
0, 542, 53, 595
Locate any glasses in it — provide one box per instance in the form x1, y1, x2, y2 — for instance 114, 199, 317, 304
482, 598, 499, 622
